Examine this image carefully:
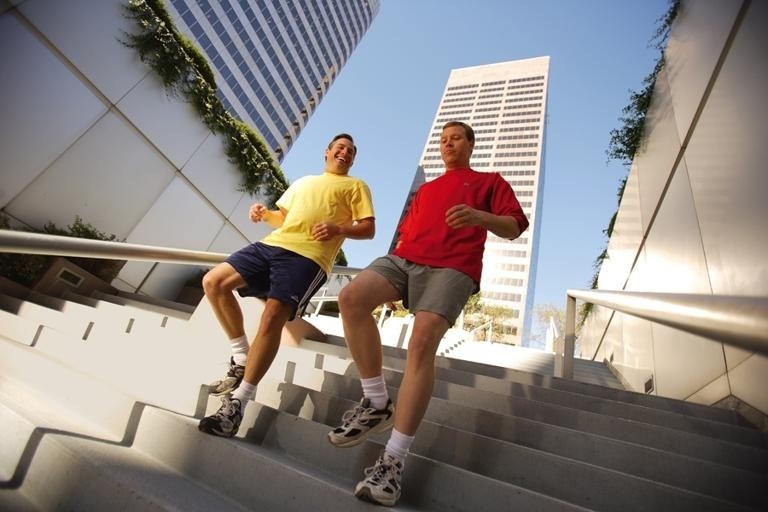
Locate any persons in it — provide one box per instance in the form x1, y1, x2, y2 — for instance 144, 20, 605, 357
327, 120, 531, 508
196, 133, 376, 439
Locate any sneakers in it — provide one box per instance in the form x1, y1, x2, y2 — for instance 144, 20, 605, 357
198, 396, 243, 437
327, 398, 396, 449
207, 355, 246, 396
354, 450, 404, 507
